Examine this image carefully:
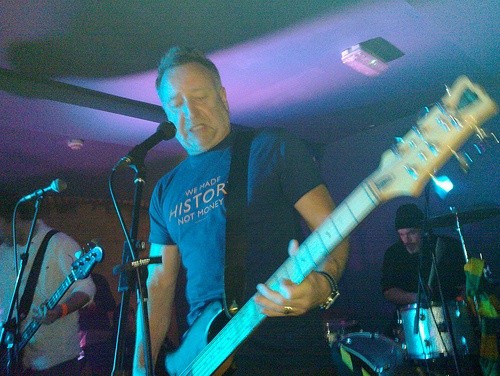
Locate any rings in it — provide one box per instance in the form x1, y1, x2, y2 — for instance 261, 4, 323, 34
284, 306, 292, 314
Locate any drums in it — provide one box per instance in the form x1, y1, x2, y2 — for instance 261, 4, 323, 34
321, 318, 364, 349
394, 299, 480, 363
326, 331, 407, 376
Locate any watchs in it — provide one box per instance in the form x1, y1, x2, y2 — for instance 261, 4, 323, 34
317, 270, 341, 311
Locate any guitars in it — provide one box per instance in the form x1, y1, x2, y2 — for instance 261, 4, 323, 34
0, 242, 106, 376
152, 72, 500, 376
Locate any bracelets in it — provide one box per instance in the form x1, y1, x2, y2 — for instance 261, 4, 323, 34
59, 303, 67, 319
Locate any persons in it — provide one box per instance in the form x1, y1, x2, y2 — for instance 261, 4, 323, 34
0, 174, 96, 376
380, 204, 485, 376
132, 46, 351, 376
79, 273, 117, 347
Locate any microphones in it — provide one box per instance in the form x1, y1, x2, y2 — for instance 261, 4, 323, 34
19, 178, 65, 203
111, 121, 177, 172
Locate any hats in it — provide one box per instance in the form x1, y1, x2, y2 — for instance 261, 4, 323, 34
395, 203, 424, 231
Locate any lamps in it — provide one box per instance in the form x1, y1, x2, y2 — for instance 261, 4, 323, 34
340, 44, 389, 78
